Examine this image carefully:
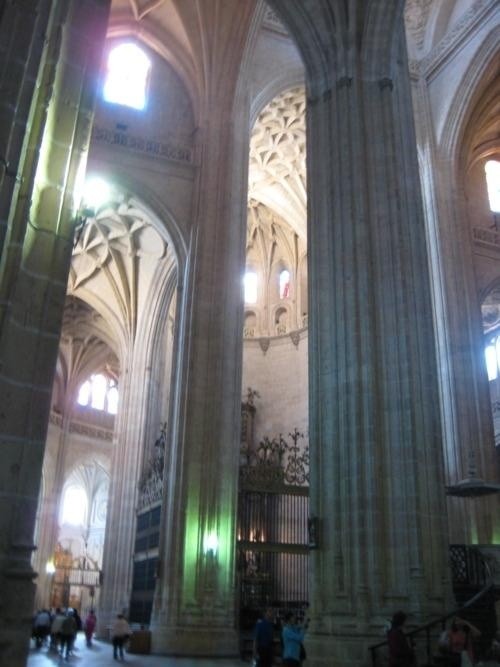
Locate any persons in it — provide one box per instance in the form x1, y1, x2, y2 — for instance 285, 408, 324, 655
384, 610, 417, 667
251, 606, 274, 667
32, 606, 96, 661
281, 610, 306, 667
111, 613, 131, 661
446, 624, 475, 667
